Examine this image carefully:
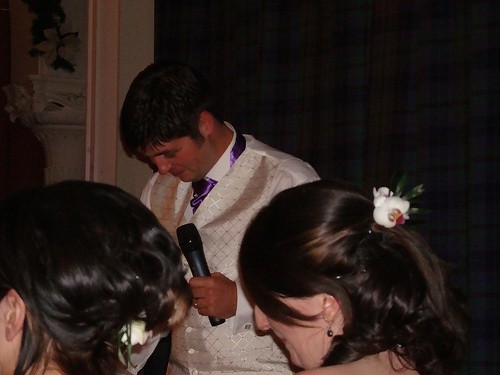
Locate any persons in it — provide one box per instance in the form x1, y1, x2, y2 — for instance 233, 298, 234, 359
119, 61, 324, 375
239, 177, 486, 375
1, 178, 193, 375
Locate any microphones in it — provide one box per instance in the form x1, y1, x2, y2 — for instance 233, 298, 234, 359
177, 223, 225, 326
